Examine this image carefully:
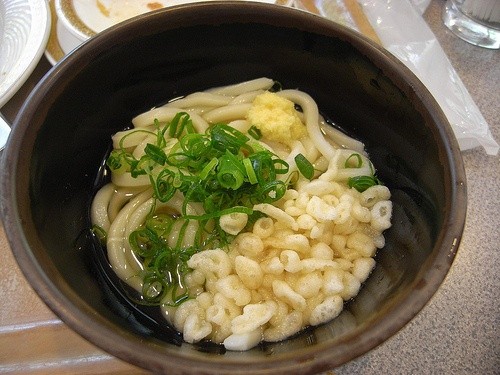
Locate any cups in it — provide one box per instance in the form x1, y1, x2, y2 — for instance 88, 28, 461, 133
441, 0, 500, 50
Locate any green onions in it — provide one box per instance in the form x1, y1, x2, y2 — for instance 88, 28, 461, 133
89, 111, 380, 306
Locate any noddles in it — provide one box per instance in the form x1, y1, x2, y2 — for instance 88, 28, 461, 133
88, 76, 393, 351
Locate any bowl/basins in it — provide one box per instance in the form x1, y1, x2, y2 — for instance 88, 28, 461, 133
0, 0, 467, 374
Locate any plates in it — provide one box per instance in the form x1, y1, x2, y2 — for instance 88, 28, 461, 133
44, 2, 294, 66
55, 1, 294, 41
0, 0, 52, 110
